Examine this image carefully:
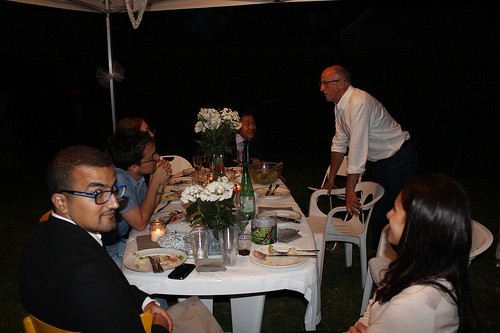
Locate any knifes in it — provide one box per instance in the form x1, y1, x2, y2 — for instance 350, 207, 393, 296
265, 184, 273, 196
148, 256, 158, 274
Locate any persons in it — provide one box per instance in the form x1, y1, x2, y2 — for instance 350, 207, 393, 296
103, 128, 172, 268
343, 175, 471, 333
13, 146, 224, 333
225, 113, 273, 166
319, 65, 409, 260
118, 118, 181, 201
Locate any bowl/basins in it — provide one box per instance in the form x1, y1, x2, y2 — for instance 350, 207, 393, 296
247, 162, 283, 185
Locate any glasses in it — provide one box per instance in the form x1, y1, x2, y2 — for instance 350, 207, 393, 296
142, 151, 159, 165
63, 185, 126, 204
319, 80, 338, 87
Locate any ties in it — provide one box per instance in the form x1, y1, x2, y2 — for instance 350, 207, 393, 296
242, 140, 248, 163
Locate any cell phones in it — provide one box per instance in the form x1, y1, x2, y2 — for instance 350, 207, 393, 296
168, 264, 195, 280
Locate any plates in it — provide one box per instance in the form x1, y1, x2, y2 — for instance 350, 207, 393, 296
257, 209, 302, 222
250, 243, 309, 266
147, 211, 181, 225
253, 186, 290, 201
161, 189, 184, 201
124, 248, 188, 273
165, 176, 194, 187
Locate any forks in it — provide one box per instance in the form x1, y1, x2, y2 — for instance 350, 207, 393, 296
155, 257, 165, 272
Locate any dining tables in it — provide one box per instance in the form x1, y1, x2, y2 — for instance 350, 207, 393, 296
120, 163, 324, 333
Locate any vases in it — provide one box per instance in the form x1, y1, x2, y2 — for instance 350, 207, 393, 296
206, 225, 226, 263
213, 153, 226, 177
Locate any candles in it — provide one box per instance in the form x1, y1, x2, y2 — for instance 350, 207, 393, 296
150, 218, 167, 242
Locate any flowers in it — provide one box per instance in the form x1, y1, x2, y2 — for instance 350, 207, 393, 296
180, 176, 246, 239
194, 107, 244, 152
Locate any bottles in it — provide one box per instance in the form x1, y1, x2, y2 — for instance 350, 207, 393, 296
237, 165, 255, 220
218, 166, 224, 177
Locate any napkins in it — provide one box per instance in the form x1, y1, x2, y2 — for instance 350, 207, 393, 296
278, 227, 302, 243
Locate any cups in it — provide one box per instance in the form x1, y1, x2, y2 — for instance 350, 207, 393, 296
239, 233, 252, 256
193, 157, 202, 168
217, 228, 238, 267
189, 229, 209, 262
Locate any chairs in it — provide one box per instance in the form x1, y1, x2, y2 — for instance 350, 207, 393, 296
305, 159, 495, 318
22, 310, 154, 333
158, 155, 193, 175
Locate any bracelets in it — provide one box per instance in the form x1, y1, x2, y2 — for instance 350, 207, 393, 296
157, 192, 164, 195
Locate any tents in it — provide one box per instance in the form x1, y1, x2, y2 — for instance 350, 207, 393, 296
7, 0, 291, 134
